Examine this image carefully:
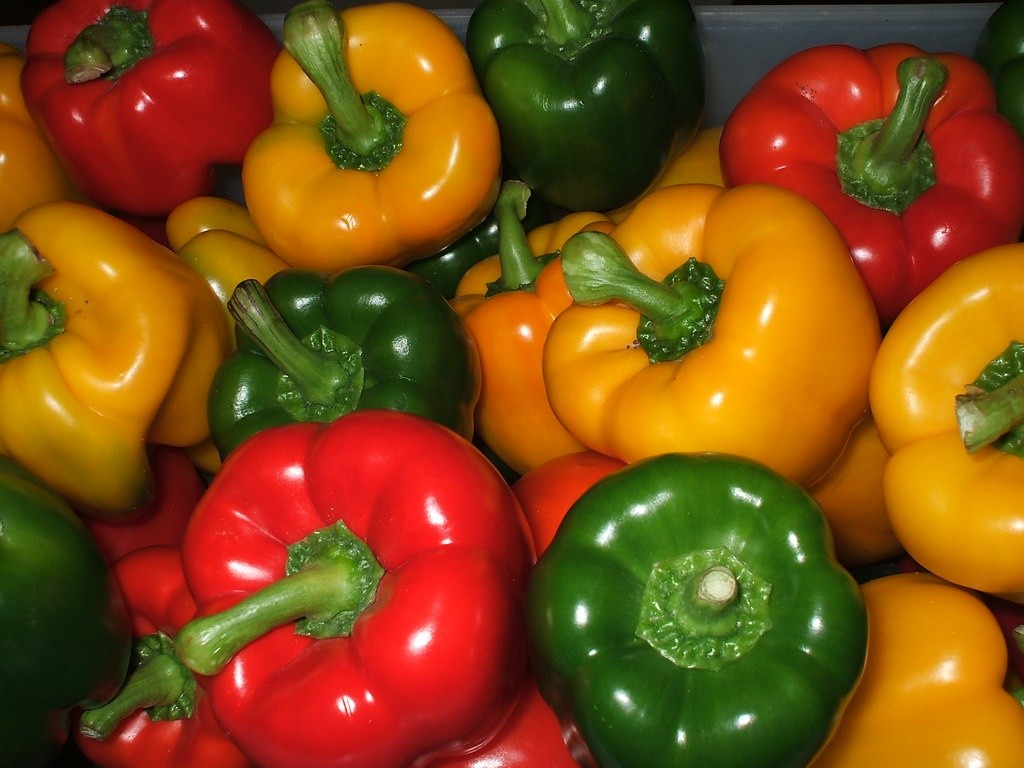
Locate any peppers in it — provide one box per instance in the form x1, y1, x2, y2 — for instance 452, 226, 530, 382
0, 0, 1024, 768
